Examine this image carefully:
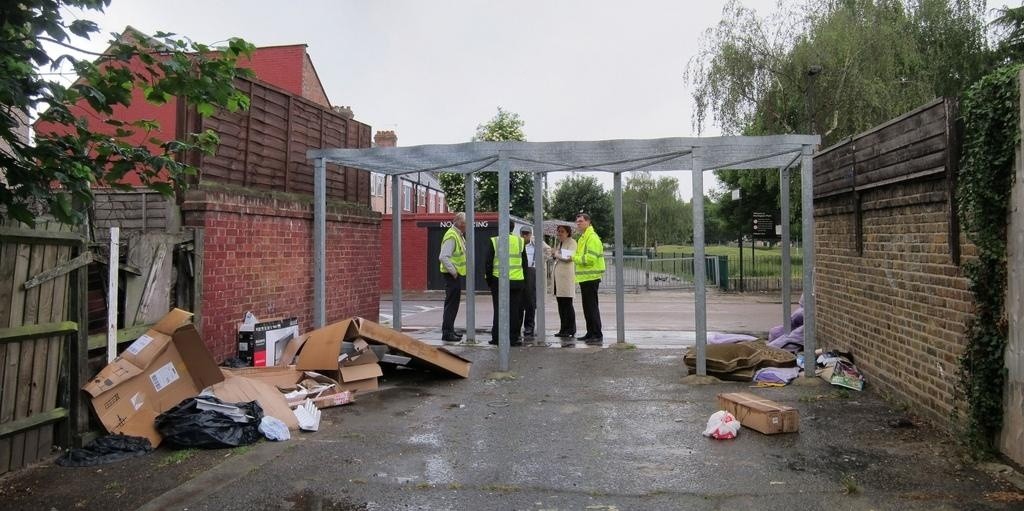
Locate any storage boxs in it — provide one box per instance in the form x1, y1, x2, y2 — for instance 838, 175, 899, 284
315, 363, 384, 393
717, 391, 800, 435
235, 316, 300, 368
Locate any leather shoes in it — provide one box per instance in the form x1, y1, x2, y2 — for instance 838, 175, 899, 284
488, 340, 498, 345
577, 332, 603, 343
442, 331, 463, 341
523, 326, 534, 335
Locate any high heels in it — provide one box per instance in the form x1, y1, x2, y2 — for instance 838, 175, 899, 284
554, 326, 575, 338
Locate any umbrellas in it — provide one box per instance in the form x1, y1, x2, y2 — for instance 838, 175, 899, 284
540, 217, 578, 246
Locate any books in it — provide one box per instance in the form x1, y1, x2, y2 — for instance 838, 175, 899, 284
826, 360, 865, 392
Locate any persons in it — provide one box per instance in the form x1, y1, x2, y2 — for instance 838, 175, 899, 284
439, 211, 471, 342
547, 223, 581, 336
516, 226, 553, 337
566, 212, 609, 345
483, 221, 529, 347
